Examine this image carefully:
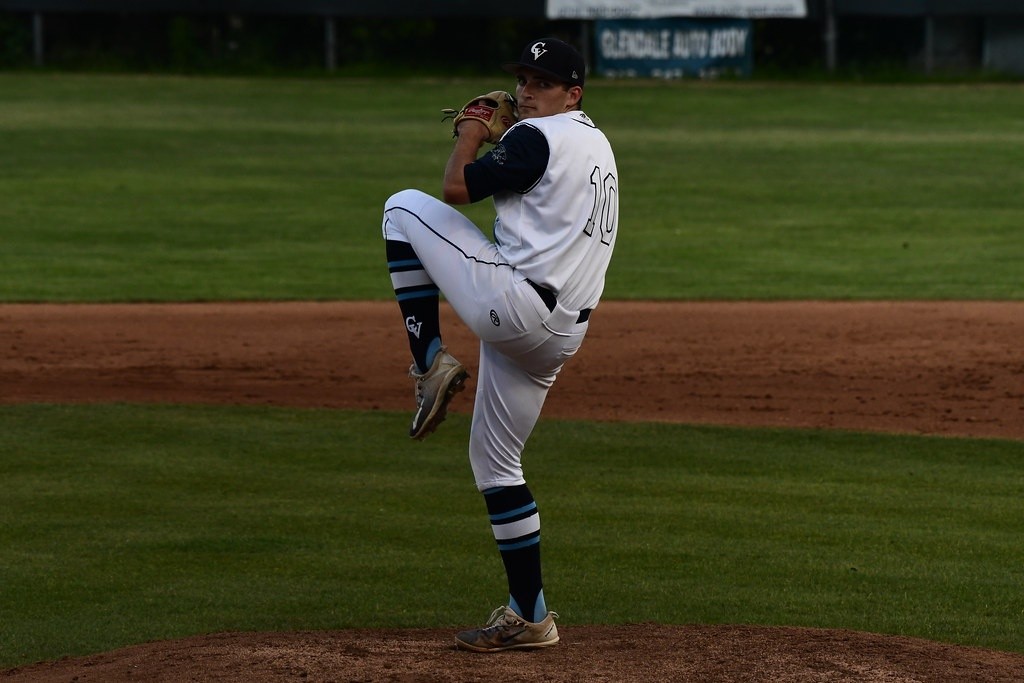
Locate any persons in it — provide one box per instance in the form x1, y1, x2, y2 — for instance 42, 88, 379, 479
381, 38, 620, 652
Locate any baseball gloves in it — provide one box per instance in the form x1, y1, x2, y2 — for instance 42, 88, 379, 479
439, 89, 520, 146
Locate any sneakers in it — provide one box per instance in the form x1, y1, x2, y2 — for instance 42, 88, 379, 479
453, 606, 561, 651
407, 344, 468, 439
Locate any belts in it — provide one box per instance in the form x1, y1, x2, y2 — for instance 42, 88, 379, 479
527, 279, 592, 325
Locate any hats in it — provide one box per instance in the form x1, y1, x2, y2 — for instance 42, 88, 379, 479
506, 37, 586, 89
